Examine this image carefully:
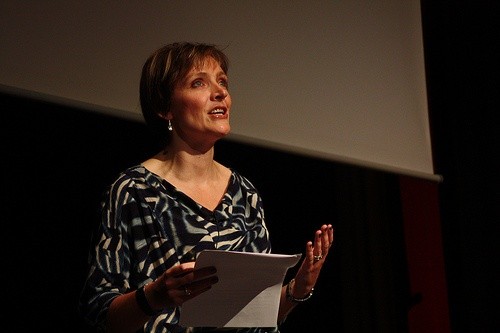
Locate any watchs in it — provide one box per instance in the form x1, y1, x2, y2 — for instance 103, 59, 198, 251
287, 278, 314, 305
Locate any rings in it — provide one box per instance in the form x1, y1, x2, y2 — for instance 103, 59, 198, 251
314, 255, 322, 261
184, 288, 192, 296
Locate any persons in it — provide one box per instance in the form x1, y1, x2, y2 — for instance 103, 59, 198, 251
82, 41, 335, 333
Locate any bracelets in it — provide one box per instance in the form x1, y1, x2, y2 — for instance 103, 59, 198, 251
136, 286, 158, 317
